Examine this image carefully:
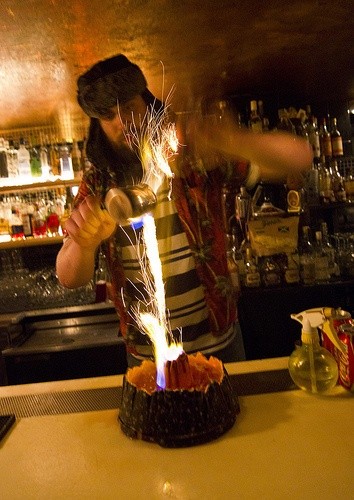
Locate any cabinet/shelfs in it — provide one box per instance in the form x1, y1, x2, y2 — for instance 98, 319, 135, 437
0, 176, 115, 320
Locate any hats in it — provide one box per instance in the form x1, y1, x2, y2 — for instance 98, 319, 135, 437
76, 54, 175, 174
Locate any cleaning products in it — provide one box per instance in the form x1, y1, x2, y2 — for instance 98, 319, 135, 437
289, 307, 346, 394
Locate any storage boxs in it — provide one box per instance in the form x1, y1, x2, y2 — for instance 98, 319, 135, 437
246, 213, 301, 256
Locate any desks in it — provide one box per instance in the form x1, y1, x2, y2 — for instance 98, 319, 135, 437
0, 357, 354, 500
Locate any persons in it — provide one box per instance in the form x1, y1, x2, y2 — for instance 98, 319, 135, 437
56, 53, 313, 369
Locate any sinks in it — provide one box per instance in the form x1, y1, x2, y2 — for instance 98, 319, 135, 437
2, 313, 124, 356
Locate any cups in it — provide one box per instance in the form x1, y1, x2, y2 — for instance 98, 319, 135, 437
1, 266, 96, 314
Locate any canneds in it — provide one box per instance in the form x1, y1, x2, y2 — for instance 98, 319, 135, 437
321, 307, 354, 392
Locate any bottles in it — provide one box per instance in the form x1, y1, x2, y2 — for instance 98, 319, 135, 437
218, 100, 354, 294
0, 134, 93, 242
